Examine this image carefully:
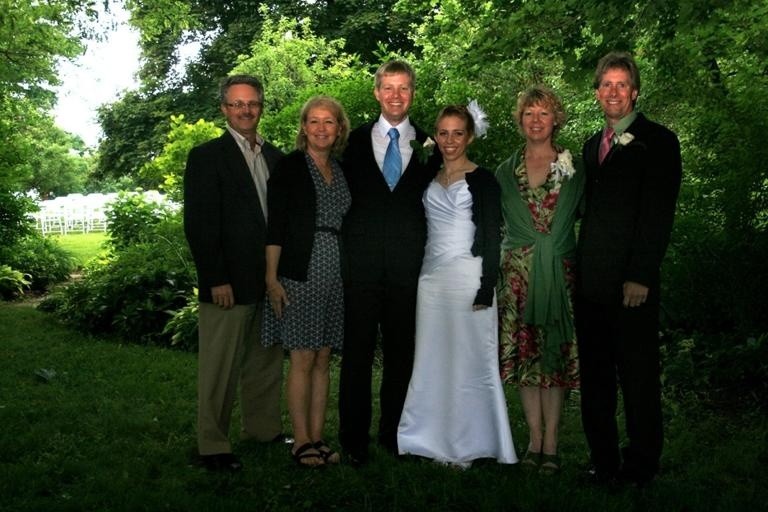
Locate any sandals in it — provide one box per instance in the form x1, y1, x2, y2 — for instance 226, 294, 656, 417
521, 450, 560, 477
290, 441, 339, 469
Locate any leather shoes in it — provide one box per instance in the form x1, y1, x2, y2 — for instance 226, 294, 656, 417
198, 453, 242, 470
432, 458, 473, 472
339, 433, 371, 464
378, 432, 398, 456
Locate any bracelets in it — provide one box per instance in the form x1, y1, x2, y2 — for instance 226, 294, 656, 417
265, 282, 282, 295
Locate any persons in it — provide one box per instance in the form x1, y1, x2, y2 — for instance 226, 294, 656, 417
337, 60, 445, 467
184, 75, 295, 472
262, 96, 352, 470
493, 84, 589, 477
571, 50, 683, 491
397, 105, 519, 471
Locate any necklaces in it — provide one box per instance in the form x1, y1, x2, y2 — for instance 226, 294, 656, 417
443, 165, 463, 187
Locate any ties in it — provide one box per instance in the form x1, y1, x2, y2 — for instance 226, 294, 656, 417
599, 128, 614, 165
383, 128, 402, 192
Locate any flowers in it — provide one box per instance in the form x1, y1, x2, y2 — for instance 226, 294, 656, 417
548, 148, 576, 180
409, 135, 437, 163
613, 127, 636, 146
